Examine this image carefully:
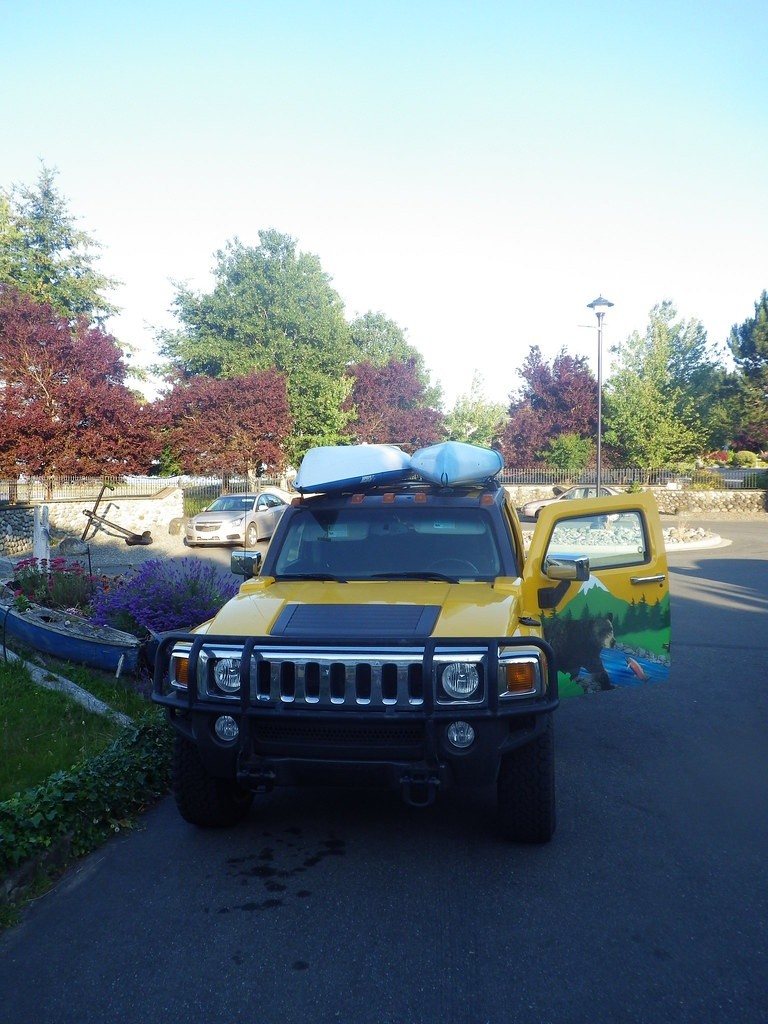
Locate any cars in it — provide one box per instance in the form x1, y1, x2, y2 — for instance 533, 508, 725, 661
518, 485, 634, 523
183, 492, 298, 549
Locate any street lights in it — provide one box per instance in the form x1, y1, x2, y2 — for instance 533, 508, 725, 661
585, 295, 616, 532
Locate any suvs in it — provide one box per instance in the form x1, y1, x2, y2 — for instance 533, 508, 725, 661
149, 440, 674, 845
